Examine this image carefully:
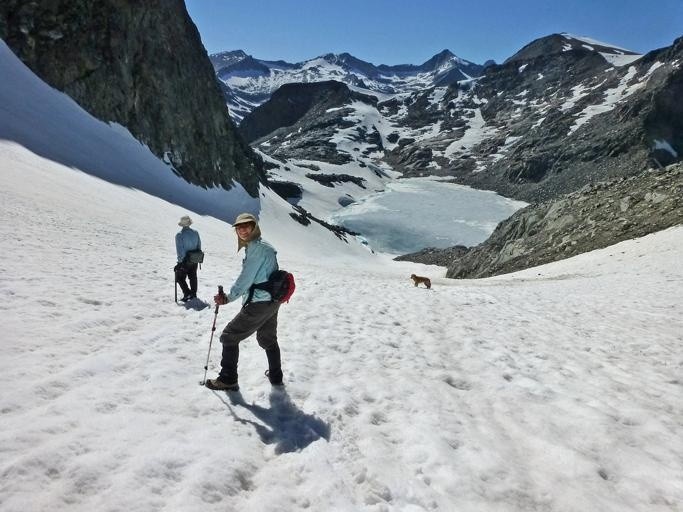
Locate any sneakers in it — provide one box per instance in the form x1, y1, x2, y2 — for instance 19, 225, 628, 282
269, 373, 283, 385
181, 292, 196, 302
205, 379, 238, 391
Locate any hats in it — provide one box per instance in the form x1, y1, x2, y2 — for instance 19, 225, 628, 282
232, 213, 257, 227
178, 216, 191, 226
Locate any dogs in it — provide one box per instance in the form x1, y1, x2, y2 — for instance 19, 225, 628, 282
411, 274, 431, 289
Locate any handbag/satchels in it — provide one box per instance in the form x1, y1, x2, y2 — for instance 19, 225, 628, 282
186, 249, 201, 266
268, 270, 295, 303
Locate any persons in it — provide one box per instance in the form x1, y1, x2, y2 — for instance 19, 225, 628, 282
204, 212, 285, 393
174, 215, 202, 302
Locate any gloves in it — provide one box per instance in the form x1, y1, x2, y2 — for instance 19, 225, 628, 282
214, 293, 228, 305
